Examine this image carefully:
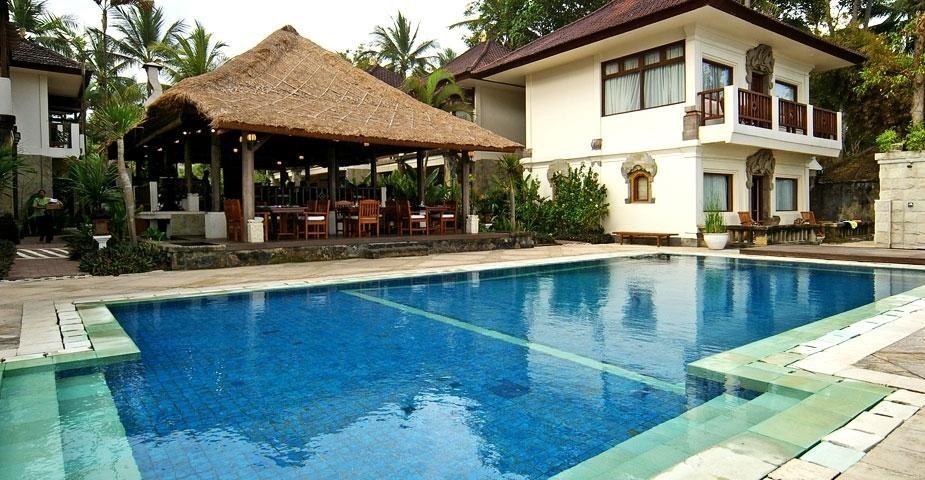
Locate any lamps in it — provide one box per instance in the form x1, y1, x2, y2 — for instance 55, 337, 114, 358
245, 132, 257, 151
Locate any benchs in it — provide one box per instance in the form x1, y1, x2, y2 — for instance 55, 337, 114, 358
612, 231, 679, 247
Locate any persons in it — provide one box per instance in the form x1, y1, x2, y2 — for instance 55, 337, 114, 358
32, 190, 54, 243
201, 170, 212, 208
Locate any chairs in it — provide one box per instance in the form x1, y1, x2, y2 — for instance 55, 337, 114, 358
738, 211, 759, 239
223, 196, 459, 242
801, 211, 824, 237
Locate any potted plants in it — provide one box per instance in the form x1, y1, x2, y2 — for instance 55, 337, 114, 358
62, 152, 131, 237
700, 190, 730, 250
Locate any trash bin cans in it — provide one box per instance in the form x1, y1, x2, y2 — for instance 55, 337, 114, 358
247, 219, 264, 243
186, 193, 200, 212
465, 214, 479, 234
